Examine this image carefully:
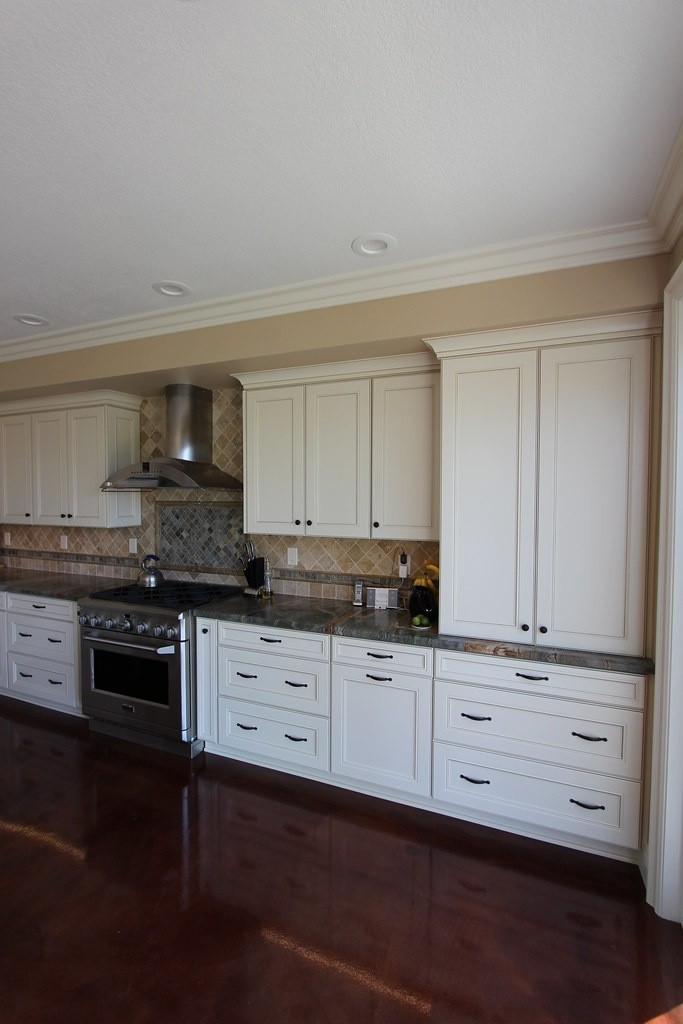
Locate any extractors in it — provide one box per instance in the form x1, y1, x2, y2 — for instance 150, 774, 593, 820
99, 384, 242, 492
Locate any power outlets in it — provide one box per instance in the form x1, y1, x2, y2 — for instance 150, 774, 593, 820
286, 547, 299, 566
59, 534, 68, 549
128, 538, 138, 553
4, 532, 11, 546
398, 554, 411, 575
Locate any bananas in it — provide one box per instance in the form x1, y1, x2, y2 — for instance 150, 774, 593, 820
414, 564, 439, 597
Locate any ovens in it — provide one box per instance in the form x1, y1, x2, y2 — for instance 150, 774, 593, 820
77, 579, 246, 759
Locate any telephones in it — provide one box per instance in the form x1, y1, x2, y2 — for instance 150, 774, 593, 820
353, 581, 363, 605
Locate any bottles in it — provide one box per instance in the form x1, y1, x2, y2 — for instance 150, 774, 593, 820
262, 561, 272, 599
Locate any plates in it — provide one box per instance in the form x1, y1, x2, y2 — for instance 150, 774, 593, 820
411, 624, 432, 628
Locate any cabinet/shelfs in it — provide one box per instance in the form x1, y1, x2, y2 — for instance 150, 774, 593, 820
330, 635, 433, 804
434, 648, 648, 852
0, 591, 9, 689
1, 404, 142, 529
439, 335, 653, 658
243, 372, 439, 542
6, 593, 79, 709
195, 618, 331, 784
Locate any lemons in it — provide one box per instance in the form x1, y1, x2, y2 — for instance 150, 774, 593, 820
411, 614, 428, 626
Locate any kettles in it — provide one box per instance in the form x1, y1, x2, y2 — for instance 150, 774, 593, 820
136, 555, 164, 587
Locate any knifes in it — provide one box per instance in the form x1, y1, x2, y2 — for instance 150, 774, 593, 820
239, 556, 247, 571
249, 540, 255, 561
244, 542, 251, 562
